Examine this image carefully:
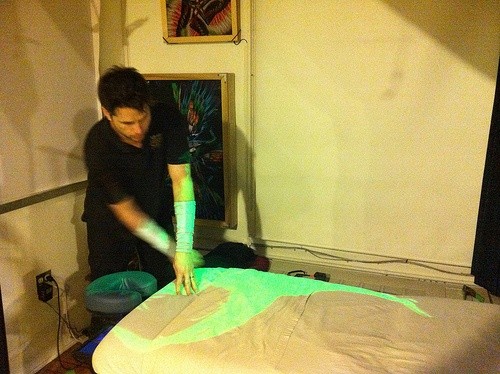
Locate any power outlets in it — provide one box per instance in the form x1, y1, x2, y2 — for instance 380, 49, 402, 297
36, 270, 54, 303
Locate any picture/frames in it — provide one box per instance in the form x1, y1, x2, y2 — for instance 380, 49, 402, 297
161, 0, 241, 44
141, 73, 237, 230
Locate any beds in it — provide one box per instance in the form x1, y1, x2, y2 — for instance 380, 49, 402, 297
92, 269, 500, 374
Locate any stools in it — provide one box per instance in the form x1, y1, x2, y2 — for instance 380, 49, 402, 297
84, 272, 158, 325
